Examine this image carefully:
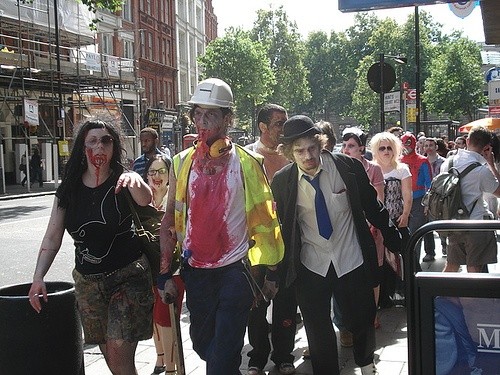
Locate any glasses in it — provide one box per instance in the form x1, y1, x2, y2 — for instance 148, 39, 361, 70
147, 168, 166, 177
85, 135, 113, 150
379, 146, 392, 151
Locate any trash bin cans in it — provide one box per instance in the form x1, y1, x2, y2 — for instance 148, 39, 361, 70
0, 280, 85, 375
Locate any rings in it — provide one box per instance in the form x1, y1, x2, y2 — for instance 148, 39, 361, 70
33, 293, 38, 298
29, 300, 31, 302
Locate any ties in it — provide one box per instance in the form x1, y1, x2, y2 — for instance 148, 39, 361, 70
303, 169, 334, 241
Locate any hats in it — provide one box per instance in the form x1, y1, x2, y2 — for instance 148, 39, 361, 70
283, 114, 321, 140
341, 126, 367, 155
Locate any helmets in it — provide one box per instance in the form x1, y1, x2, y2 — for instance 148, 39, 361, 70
187, 78, 234, 108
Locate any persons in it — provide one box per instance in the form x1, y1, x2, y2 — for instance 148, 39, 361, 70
25, 148, 43, 188
20, 149, 29, 187
27, 119, 163, 374
27, 116, 500, 375
241, 104, 304, 374
154, 74, 287, 375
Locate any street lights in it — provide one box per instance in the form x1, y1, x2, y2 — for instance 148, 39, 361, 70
379, 50, 408, 135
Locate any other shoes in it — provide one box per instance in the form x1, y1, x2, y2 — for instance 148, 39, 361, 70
153, 353, 166, 374
248, 366, 259, 375
423, 251, 435, 262
302, 349, 310, 357
442, 244, 447, 255
279, 362, 295, 374
340, 329, 353, 347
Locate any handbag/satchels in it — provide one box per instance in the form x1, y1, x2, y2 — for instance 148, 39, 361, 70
142, 211, 181, 290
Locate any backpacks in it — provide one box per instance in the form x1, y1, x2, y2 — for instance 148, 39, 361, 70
427, 157, 482, 238
